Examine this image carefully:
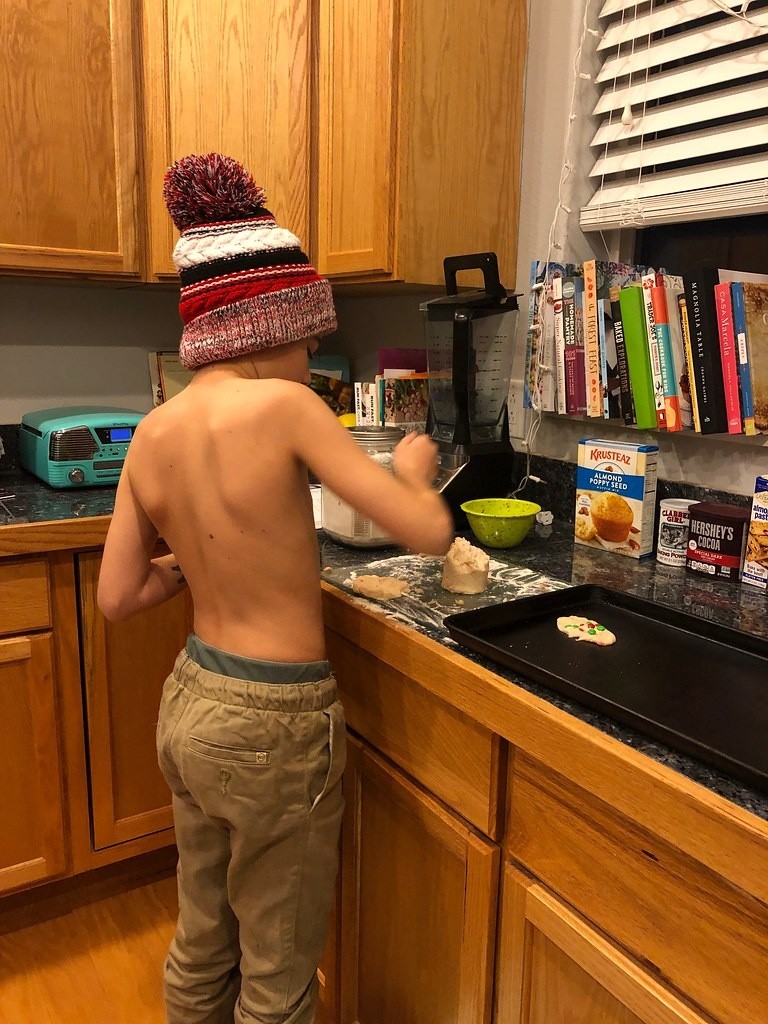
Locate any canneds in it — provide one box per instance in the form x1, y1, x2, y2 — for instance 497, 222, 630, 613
656, 498, 702, 567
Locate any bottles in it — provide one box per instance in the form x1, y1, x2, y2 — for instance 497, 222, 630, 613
319, 423, 411, 547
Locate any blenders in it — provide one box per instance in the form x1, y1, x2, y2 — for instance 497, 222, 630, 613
397, 255, 525, 536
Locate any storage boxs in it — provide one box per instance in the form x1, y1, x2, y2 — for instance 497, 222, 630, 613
574, 439, 768, 588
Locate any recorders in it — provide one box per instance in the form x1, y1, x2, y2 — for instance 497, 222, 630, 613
18, 406, 148, 487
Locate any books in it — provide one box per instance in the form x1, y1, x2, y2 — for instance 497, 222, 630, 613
523, 260, 768, 435
303, 346, 452, 434
149, 351, 196, 408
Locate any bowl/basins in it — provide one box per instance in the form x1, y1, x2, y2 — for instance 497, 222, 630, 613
459, 498, 542, 548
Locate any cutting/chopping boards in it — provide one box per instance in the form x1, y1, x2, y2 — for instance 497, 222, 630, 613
320, 550, 575, 638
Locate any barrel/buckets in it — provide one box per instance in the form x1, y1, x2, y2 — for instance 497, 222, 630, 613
657, 498, 699, 568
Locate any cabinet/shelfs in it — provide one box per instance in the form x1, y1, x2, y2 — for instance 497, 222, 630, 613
315, 627, 506, 1024
491, 736, 767, 1024
0, 548, 69, 898
73, 548, 194, 853
0, 1, 531, 295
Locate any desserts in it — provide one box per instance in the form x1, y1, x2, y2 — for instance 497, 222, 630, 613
589, 491, 634, 542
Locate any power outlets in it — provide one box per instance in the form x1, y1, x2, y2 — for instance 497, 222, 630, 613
499, 380, 526, 441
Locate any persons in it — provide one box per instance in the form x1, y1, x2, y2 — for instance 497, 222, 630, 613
98, 154, 452, 1024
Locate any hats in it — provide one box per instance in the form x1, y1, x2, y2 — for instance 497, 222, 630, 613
162, 152, 338, 372
